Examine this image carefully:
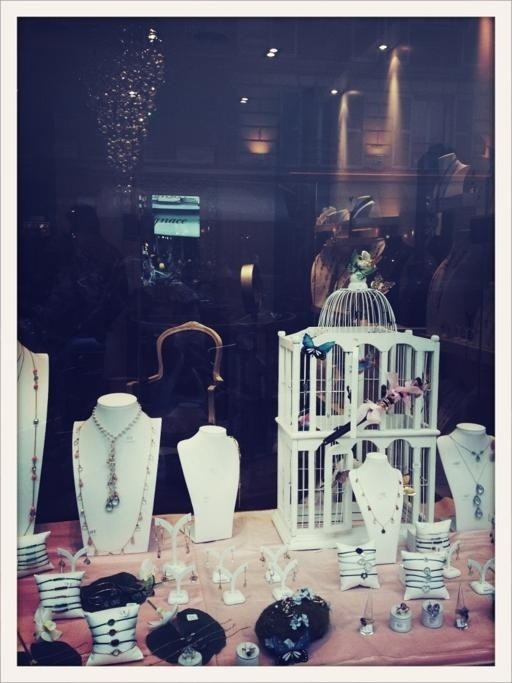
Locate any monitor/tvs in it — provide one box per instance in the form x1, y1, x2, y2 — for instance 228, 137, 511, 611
152, 195, 201, 240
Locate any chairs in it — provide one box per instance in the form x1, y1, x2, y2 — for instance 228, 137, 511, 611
124, 320, 225, 446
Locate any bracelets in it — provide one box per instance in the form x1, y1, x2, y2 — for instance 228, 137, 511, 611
338, 548, 378, 579
18, 543, 49, 571
39, 576, 85, 616
402, 556, 449, 593
89, 613, 138, 656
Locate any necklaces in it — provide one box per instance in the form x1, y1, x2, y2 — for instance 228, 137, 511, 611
18, 344, 38, 537
92, 405, 142, 512
356, 470, 402, 534
450, 435, 492, 519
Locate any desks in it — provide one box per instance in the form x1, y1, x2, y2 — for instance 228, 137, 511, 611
17, 495, 496, 666
